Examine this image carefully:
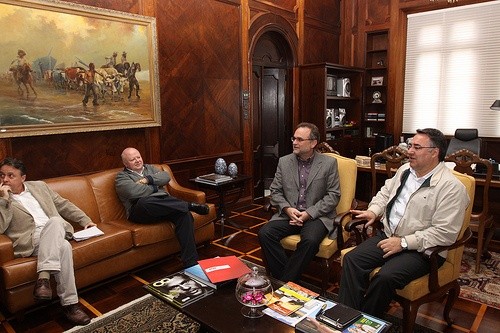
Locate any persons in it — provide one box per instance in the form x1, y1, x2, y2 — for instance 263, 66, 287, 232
257, 123, 341, 284
0, 155, 97, 325
339, 129, 471, 317
115, 147, 209, 270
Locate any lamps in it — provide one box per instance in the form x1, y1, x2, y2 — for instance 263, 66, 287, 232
490, 100, 500, 111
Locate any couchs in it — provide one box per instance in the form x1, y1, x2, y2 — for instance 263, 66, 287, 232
0, 162, 216, 313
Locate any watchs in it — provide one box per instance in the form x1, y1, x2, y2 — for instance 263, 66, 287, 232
401, 237, 408, 251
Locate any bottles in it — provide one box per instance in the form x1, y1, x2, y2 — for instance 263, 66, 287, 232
228, 163, 238, 175
215, 158, 227, 175
235, 266, 274, 319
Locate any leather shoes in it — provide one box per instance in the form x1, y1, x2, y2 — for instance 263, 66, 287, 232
34, 278, 52, 299
64, 303, 90, 325
189, 203, 209, 216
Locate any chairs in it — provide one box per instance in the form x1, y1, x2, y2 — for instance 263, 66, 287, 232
278, 128, 499, 333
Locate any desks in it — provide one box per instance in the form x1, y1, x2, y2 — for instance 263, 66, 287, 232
352, 161, 500, 188
142, 258, 393, 333
189, 173, 251, 236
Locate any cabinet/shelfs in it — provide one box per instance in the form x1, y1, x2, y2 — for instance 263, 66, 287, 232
300, 48, 393, 155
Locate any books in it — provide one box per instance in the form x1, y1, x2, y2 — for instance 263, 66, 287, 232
142, 273, 215, 310
366, 113, 386, 121
261, 280, 385, 333
185, 255, 253, 289
325, 133, 335, 140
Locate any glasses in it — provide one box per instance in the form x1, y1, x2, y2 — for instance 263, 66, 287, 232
407, 144, 436, 151
291, 136, 318, 143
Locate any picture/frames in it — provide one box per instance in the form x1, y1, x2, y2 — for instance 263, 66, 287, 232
0, 0, 162, 140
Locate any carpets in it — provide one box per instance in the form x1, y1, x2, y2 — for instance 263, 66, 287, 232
448, 246, 500, 309
62, 257, 212, 333
